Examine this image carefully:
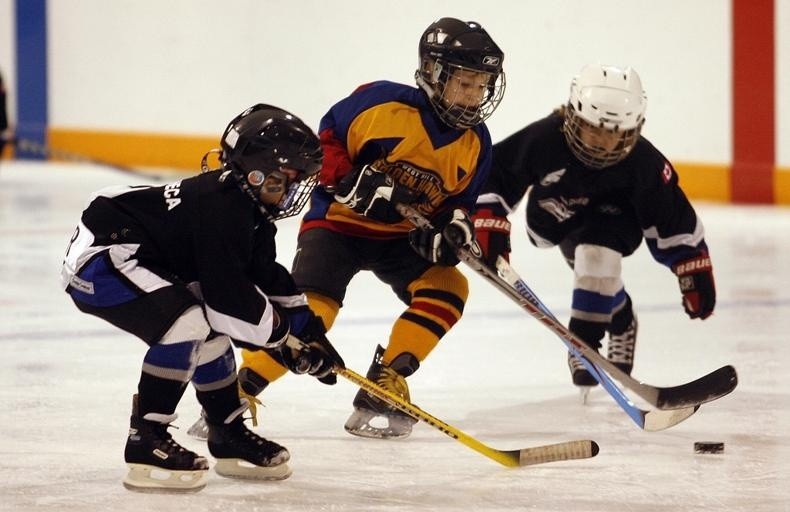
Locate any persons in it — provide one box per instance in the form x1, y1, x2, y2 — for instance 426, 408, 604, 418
58, 104, 345, 471
470, 60, 717, 386
187, 17, 506, 439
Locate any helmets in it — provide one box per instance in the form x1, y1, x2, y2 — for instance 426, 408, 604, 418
220, 103, 323, 203
563, 63, 647, 151
415, 17, 505, 100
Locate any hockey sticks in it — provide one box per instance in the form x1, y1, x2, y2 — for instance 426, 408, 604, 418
496, 255, 700, 431
397, 205, 737, 411
286, 335, 599, 467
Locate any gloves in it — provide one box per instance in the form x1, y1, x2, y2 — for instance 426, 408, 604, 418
284, 316, 345, 386
673, 244, 717, 323
410, 209, 470, 266
472, 199, 513, 276
335, 164, 422, 224
233, 297, 290, 353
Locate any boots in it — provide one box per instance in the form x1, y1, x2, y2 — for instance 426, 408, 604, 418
567, 329, 605, 386
354, 346, 420, 424
122, 393, 209, 470
607, 313, 638, 376
199, 387, 290, 465
